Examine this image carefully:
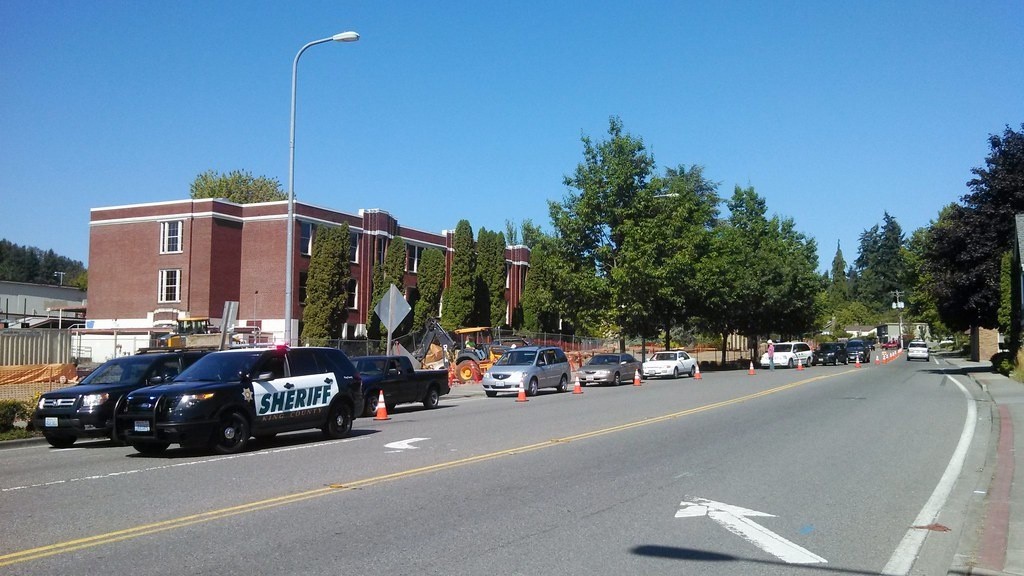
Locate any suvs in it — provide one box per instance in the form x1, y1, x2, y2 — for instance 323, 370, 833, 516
483, 347, 571, 399
111, 346, 366, 455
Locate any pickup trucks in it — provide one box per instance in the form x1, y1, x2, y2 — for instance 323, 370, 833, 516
347, 355, 451, 408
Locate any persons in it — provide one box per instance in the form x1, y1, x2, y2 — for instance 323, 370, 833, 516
767, 338, 777, 374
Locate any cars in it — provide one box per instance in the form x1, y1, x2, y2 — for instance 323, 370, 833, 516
761, 340, 813, 367
811, 342, 849, 366
906, 340, 932, 361
575, 352, 643, 385
29, 350, 205, 447
883, 340, 898, 348
864, 341, 877, 351
898, 334, 913, 347
641, 349, 698, 378
843, 338, 870, 365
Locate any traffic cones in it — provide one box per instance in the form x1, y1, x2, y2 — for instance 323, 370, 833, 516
372, 389, 392, 421
692, 363, 702, 380
514, 377, 529, 403
632, 367, 642, 387
573, 371, 585, 395
855, 353, 861, 367
873, 346, 904, 366
748, 359, 757, 376
794, 357, 805, 373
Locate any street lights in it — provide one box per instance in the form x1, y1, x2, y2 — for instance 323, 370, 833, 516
889, 289, 906, 348
281, 28, 362, 346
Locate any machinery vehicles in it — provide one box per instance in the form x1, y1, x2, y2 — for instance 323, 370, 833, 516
158, 318, 242, 351
412, 317, 514, 382
